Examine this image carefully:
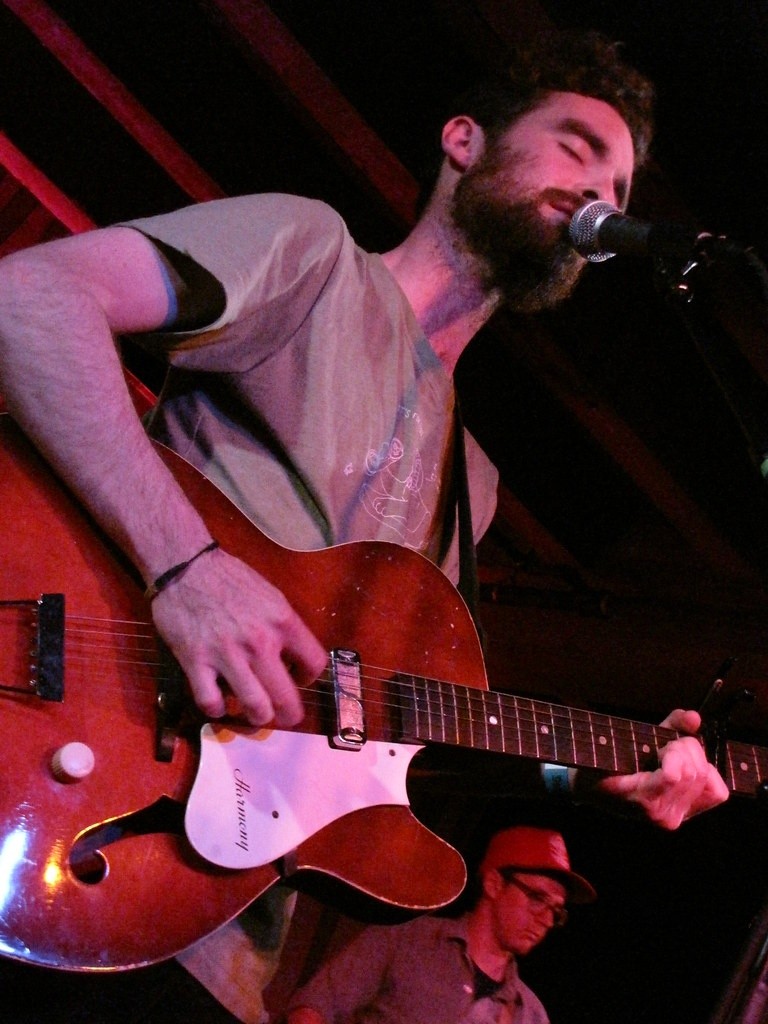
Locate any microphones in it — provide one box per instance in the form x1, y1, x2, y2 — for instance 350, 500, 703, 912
568, 200, 758, 265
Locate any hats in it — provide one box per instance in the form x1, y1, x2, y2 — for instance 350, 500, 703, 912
482, 827, 596, 897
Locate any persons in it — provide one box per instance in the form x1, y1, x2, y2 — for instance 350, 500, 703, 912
285, 827, 596, 1024
0, 27, 728, 1024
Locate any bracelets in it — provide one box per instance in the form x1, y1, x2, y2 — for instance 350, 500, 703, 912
143, 538, 219, 598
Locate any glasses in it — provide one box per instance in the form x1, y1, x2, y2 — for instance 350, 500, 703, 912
501, 872, 567, 927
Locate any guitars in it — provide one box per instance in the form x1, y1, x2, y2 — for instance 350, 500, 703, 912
0, 409, 768, 983
